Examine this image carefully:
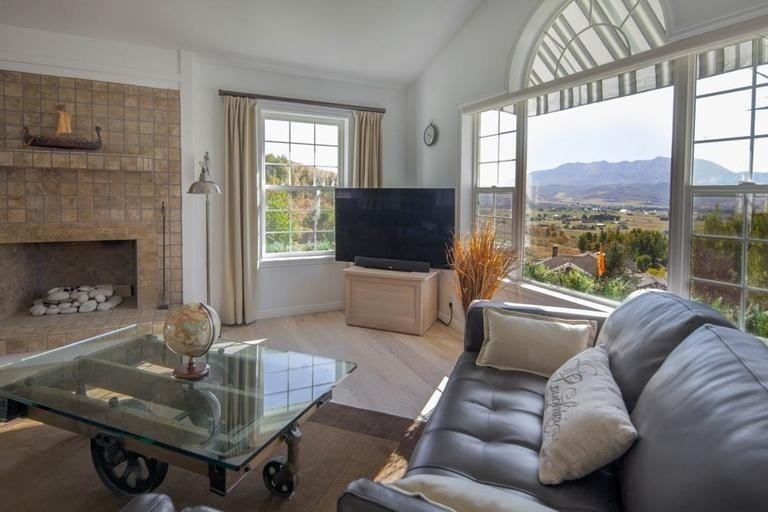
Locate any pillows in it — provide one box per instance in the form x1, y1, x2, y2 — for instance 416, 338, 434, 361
375, 469, 559, 512
536, 342, 639, 486
478, 303, 598, 378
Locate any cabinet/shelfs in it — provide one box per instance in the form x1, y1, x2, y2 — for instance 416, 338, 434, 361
342, 264, 440, 336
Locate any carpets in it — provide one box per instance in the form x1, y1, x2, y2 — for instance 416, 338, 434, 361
0, 387, 426, 512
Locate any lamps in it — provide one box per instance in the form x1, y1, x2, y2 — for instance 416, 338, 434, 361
187, 151, 223, 308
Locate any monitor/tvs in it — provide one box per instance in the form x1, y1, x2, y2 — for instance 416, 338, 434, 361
333, 186, 457, 274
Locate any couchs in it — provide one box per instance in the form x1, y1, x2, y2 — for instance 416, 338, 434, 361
334, 284, 767, 511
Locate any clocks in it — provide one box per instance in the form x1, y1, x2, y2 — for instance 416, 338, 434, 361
424, 123, 437, 147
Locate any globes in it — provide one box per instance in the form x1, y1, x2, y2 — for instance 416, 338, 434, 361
163, 303, 221, 379
